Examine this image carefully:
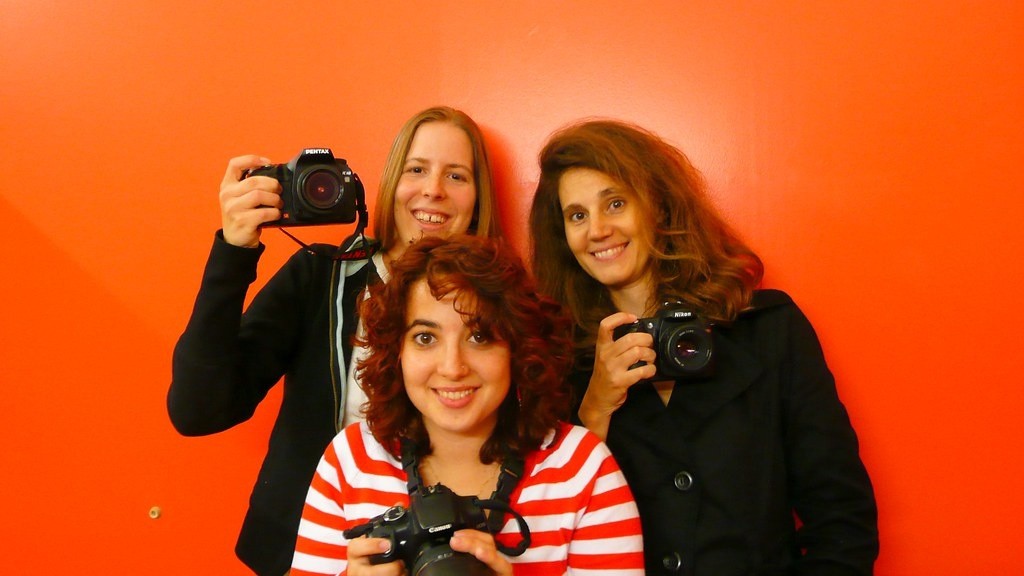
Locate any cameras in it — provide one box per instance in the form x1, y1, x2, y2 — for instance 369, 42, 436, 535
613, 304, 714, 384
248, 148, 358, 227
366, 484, 494, 576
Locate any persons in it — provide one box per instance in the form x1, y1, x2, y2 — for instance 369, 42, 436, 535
166, 106, 514, 576
525, 118, 879, 576
289, 233, 644, 576
